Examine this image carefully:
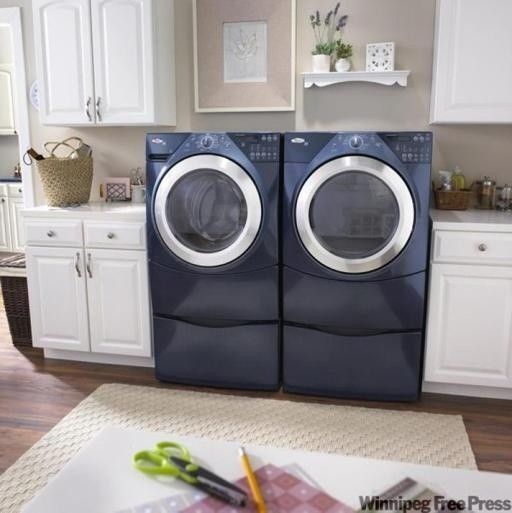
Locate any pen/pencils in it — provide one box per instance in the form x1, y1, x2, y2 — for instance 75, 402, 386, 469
170, 453, 250, 498
238, 446, 269, 513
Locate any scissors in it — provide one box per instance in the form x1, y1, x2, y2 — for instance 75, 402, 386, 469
131, 440, 245, 506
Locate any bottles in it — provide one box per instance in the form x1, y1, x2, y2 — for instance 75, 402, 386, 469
504, 183, 512, 210
451, 165, 465, 191
495, 186, 506, 211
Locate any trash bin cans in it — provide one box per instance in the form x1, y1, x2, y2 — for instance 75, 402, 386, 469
0, 252, 32, 346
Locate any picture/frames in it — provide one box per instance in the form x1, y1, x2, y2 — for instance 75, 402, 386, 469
192, 0, 296, 113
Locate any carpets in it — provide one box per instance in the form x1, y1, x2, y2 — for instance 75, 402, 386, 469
1, 382, 480, 513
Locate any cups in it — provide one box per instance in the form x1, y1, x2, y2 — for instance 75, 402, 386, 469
131, 184, 146, 203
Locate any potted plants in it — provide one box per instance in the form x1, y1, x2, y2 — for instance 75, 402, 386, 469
309, 2, 348, 73
335, 38, 352, 72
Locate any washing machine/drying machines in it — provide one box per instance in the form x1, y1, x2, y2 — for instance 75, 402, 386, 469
282, 131, 433, 401
145, 132, 282, 392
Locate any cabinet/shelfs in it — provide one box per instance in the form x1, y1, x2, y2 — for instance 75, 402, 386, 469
32, 0, 177, 129
21, 198, 156, 369
428, 1, 512, 126
421, 207, 512, 401
0, 182, 26, 254
0, 24, 18, 135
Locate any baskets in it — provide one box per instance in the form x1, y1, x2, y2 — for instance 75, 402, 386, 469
432, 179, 476, 210
1, 275, 31, 346
35, 138, 93, 207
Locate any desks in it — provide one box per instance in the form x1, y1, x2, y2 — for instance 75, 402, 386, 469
19, 423, 512, 513
